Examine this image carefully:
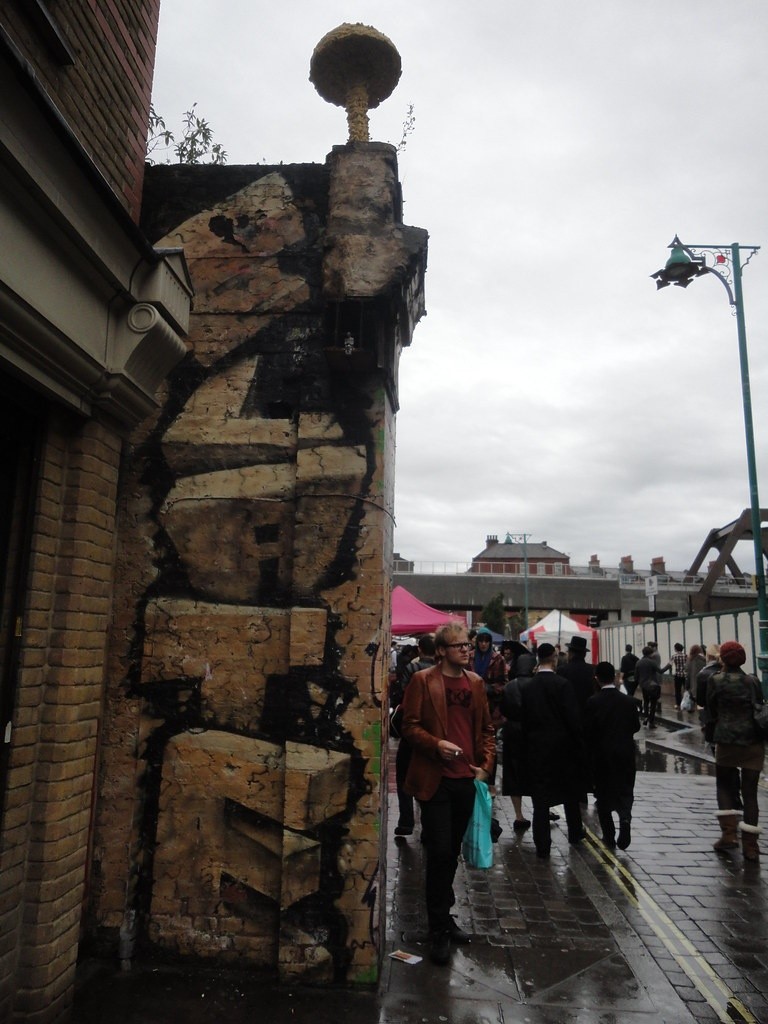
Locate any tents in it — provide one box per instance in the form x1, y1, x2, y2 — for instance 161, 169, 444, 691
390, 583, 467, 635
519, 609, 598, 665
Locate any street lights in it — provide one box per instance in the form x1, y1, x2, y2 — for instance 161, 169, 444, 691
650, 234, 768, 702
503, 533, 533, 630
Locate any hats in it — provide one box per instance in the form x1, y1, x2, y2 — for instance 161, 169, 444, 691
564, 636, 590, 652
705, 644, 722, 658
720, 642, 746, 666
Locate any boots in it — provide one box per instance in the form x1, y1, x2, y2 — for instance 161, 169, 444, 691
713, 810, 743, 849
740, 820, 763, 859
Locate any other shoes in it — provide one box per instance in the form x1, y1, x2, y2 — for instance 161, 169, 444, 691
570, 827, 587, 846
394, 826, 414, 835
536, 851, 551, 860
674, 705, 678, 708
427, 931, 450, 963
642, 716, 649, 726
513, 819, 531, 832
616, 820, 631, 850
549, 813, 560, 821
648, 724, 657, 728
603, 837, 617, 848
444, 921, 470, 942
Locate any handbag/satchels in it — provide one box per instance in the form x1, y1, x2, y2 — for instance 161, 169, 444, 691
681, 691, 692, 711
619, 683, 628, 695
463, 778, 494, 870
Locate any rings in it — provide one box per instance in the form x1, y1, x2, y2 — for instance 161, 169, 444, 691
454, 752, 459, 755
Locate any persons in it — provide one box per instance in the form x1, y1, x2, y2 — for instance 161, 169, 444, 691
401, 622, 497, 939
647, 642, 662, 712
696, 641, 768, 858
557, 636, 597, 805
684, 644, 707, 701
465, 628, 512, 799
619, 644, 643, 713
500, 642, 586, 858
390, 637, 423, 842
635, 647, 672, 729
584, 662, 641, 851
661, 642, 688, 709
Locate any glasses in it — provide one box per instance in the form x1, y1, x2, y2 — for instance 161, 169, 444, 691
443, 642, 471, 651
